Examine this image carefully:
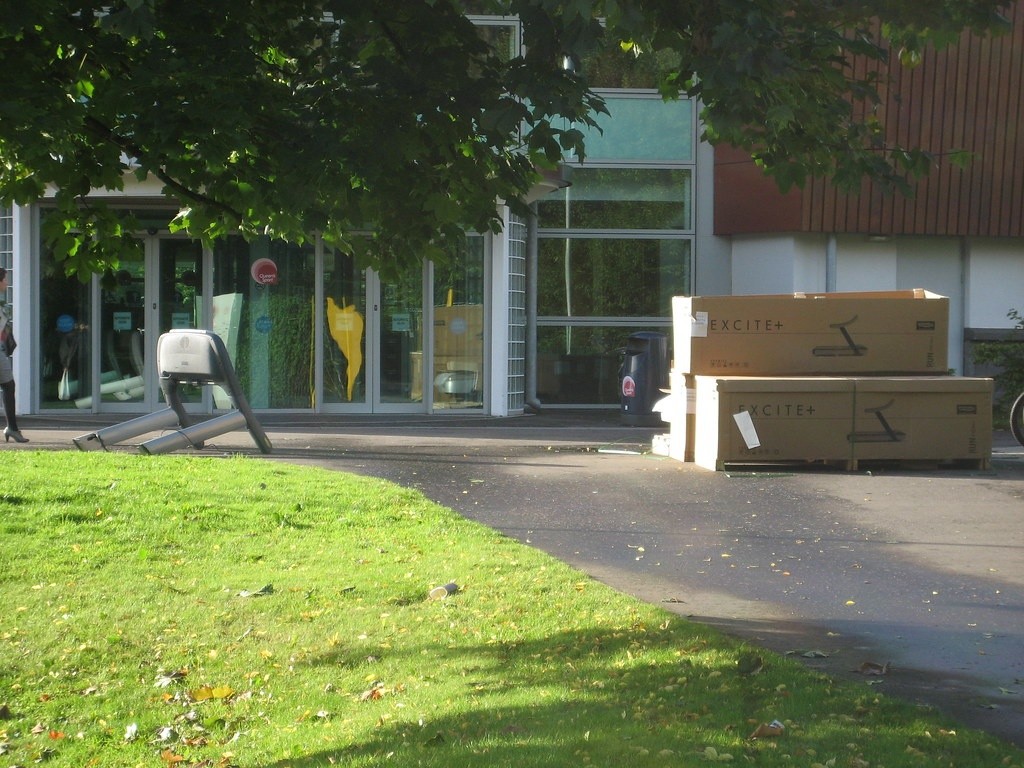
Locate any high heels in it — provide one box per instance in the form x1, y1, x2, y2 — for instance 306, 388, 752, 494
3, 427, 29, 443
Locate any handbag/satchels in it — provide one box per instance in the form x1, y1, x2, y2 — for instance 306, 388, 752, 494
57, 368, 71, 401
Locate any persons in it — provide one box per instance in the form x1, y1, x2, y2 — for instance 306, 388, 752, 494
0, 268, 29, 443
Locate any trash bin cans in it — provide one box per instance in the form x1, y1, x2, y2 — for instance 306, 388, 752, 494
619, 331, 671, 427
